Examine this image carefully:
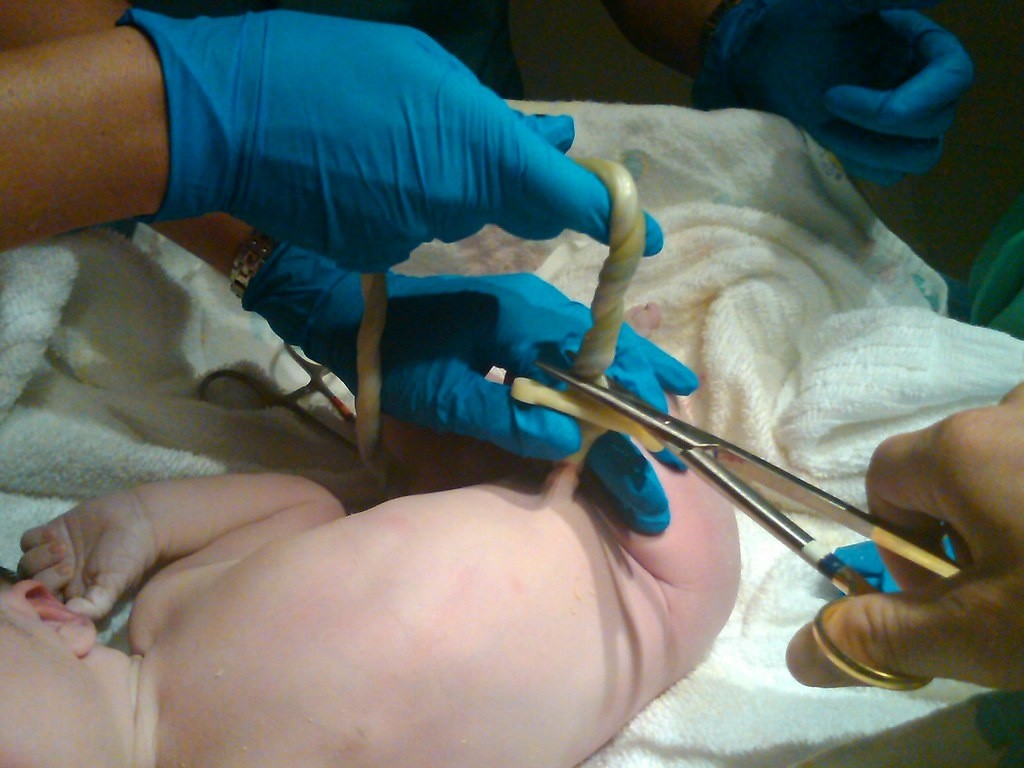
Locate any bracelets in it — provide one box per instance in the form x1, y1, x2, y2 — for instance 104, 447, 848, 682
699, 0, 737, 69
228, 226, 280, 300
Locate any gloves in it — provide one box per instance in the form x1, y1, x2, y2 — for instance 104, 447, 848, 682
116, 7, 666, 275
825, 532, 956, 598
690, 0, 974, 189
240, 240, 702, 533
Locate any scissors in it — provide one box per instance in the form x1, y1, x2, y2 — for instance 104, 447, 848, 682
199, 340, 357, 453
531, 348, 975, 692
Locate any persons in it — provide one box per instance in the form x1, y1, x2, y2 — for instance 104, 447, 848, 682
0, 0, 1024, 768
